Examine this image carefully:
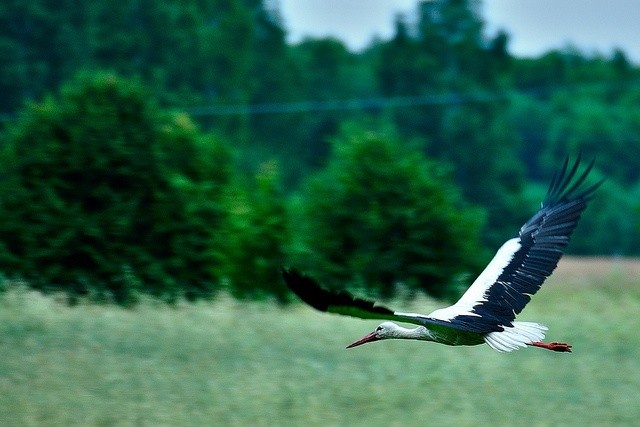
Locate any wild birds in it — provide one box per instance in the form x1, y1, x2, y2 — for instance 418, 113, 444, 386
280, 150, 608, 352
